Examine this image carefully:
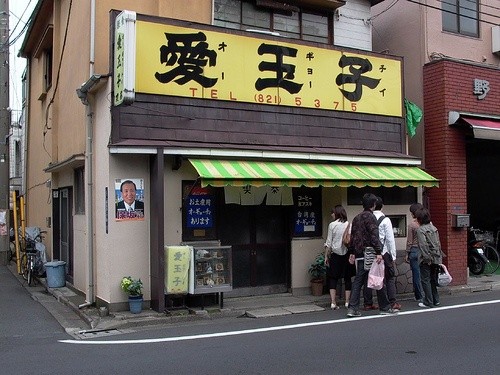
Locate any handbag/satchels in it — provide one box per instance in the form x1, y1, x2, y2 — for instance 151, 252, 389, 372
438, 264, 452, 286
367, 259, 385, 290
342, 223, 350, 248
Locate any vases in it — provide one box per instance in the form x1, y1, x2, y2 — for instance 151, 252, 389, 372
129, 299, 142, 314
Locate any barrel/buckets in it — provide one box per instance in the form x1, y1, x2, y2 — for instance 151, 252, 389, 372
45, 261, 67, 288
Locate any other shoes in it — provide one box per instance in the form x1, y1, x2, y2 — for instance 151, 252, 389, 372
418, 302, 428, 307
392, 303, 401, 309
365, 305, 379, 310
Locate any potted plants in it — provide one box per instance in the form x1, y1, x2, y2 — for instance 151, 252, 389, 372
308, 253, 327, 296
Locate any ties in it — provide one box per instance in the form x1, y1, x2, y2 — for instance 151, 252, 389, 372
128, 207, 133, 212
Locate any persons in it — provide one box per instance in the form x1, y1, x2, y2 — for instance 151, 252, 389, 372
115, 181, 144, 218
325, 194, 444, 316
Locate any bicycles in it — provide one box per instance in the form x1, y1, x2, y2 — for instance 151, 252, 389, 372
12, 230, 47, 287
480, 230, 500, 275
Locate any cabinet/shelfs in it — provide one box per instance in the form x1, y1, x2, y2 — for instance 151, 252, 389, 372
188, 246, 233, 294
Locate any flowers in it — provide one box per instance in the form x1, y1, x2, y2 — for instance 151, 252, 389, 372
120, 276, 143, 298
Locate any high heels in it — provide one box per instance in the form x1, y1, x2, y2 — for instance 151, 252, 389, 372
345, 303, 348, 309
331, 304, 340, 310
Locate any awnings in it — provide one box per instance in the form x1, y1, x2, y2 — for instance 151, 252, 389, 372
187, 158, 441, 189
458, 116, 500, 141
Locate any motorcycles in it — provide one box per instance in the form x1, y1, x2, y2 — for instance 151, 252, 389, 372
468, 225, 490, 276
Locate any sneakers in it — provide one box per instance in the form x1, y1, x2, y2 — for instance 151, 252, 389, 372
347, 309, 362, 316
380, 308, 399, 315
